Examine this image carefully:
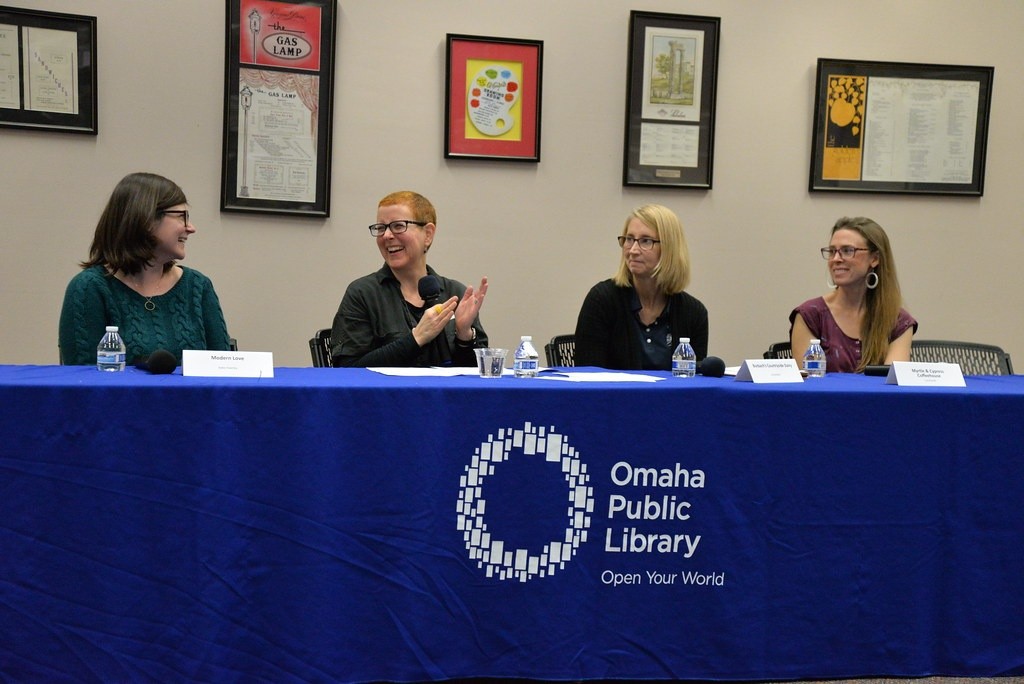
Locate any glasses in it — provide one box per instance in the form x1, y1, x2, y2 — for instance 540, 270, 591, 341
821, 245, 872, 260
369, 220, 426, 237
618, 236, 660, 250
158, 210, 189, 227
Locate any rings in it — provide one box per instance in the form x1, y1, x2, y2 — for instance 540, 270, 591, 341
474, 299, 478, 304
435, 306, 442, 315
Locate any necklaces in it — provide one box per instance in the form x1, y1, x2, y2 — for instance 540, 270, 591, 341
130, 279, 161, 310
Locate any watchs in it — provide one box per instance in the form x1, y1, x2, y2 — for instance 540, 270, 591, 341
454, 327, 477, 346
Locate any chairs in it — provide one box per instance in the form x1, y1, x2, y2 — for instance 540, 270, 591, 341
307, 327, 1013, 375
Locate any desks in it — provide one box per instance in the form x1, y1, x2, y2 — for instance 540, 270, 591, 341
1, 368, 1023, 683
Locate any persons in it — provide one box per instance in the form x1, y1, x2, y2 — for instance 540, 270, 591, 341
329, 190, 489, 368
789, 216, 917, 373
574, 202, 709, 372
56, 172, 232, 367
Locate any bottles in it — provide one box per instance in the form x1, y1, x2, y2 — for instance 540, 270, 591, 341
672, 338, 696, 377
513, 336, 539, 378
802, 339, 826, 377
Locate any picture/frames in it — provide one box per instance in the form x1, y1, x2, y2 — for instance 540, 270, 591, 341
620, 10, 721, 189
803, 57, 994, 198
440, 33, 544, 163
221, 0, 337, 219
0, 6, 100, 136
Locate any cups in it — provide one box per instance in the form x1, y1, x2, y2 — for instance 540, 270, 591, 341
97, 326, 126, 371
473, 348, 508, 378
